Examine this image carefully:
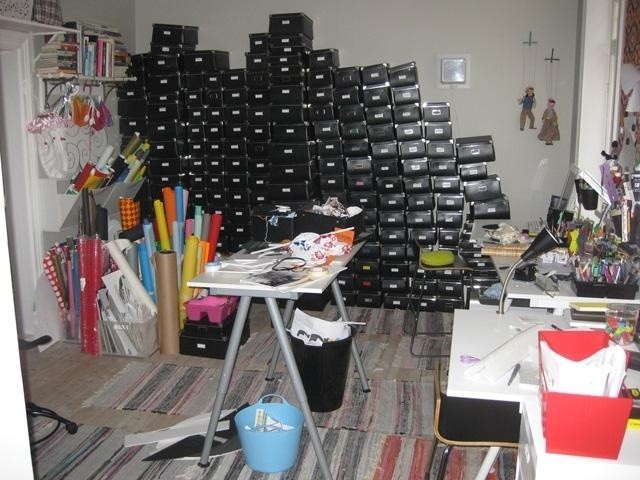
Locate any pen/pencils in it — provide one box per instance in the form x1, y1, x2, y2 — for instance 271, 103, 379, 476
572, 253, 640, 285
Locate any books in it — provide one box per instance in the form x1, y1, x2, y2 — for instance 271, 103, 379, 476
480, 243, 528, 256
35, 34, 129, 78
240, 271, 308, 288
564, 302, 607, 329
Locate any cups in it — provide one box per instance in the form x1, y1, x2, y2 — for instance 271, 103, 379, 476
606, 303, 639, 345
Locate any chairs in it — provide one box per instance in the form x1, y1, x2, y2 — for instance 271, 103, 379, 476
402, 237, 474, 357
424, 358, 519, 479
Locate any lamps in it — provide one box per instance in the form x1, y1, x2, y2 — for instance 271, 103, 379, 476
498, 227, 560, 315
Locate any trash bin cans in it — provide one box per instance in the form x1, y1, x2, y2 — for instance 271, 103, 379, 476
286, 324, 356, 412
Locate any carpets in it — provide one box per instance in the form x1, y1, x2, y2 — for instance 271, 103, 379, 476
30, 301, 518, 479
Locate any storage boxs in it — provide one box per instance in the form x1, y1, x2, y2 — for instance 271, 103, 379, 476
56, 307, 81, 344
96, 313, 160, 359
184, 312, 246, 337
183, 295, 238, 323
537, 328, 634, 461
179, 324, 250, 360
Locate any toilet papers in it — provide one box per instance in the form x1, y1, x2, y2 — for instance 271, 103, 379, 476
152, 250, 180, 355
464, 323, 539, 385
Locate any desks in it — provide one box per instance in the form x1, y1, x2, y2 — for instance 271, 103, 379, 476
446, 308, 640, 480
468, 219, 640, 314
186, 239, 372, 479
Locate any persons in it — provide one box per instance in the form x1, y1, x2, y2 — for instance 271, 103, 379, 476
537, 99, 560, 145
517, 88, 537, 131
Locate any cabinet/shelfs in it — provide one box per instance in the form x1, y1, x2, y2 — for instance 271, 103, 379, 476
0, 15, 138, 111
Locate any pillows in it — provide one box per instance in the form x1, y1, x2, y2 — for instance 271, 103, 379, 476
421, 250, 455, 267
